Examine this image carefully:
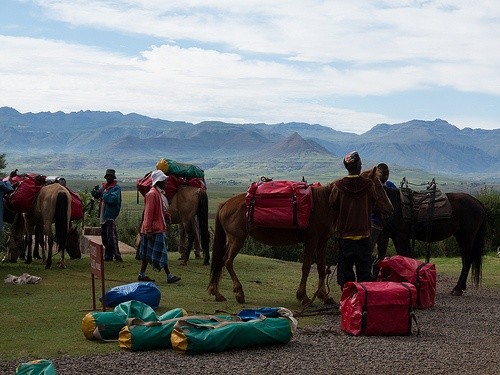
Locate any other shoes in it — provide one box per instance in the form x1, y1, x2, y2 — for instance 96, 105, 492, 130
137, 275, 155, 282
114, 258, 123, 263
167, 275, 182, 283
104, 257, 113, 262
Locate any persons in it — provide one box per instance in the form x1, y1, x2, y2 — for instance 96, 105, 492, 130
0, 179, 14, 244
92, 169, 123, 262
327, 152, 400, 293
138, 170, 181, 283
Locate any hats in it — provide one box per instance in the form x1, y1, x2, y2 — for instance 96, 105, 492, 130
104, 169, 116, 179
344, 151, 360, 163
151, 169, 168, 186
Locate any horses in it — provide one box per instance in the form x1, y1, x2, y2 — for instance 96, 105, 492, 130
205, 166, 395, 308
135, 186, 210, 266
5, 182, 72, 269
377, 186, 488, 296
